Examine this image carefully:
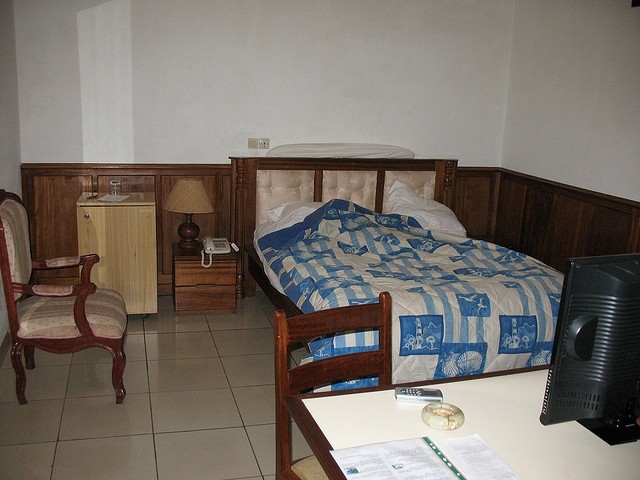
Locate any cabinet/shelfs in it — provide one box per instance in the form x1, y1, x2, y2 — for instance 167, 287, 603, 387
76, 192, 158, 318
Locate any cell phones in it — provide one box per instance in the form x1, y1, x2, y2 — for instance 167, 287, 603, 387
395, 386, 444, 405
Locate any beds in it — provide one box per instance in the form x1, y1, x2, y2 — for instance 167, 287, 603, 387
227, 155, 564, 391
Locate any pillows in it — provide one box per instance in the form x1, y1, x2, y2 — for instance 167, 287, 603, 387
389, 179, 466, 235
265, 201, 327, 231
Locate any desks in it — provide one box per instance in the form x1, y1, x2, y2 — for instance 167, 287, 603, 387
286, 364, 640, 480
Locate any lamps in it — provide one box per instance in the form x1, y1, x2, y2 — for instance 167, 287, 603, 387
162, 179, 214, 251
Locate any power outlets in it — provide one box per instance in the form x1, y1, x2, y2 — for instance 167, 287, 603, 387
259, 139, 270, 149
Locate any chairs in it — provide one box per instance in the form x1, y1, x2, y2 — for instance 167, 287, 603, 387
1, 189, 129, 404
275, 292, 392, 479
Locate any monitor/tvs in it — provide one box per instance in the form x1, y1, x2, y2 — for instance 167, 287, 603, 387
539, 253, 640, 446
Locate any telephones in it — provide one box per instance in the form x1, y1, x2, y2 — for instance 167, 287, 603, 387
201, 236, 231, 267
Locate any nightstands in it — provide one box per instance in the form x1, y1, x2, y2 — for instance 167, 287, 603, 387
172, 241, 237, 312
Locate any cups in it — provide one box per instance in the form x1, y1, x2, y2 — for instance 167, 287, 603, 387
109, 181, 121, 201
